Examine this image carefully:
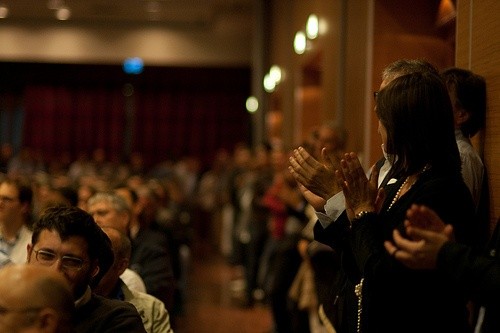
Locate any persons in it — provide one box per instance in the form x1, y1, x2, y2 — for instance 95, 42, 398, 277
288, 57, 448, 333
384, 200, 500, 333
1, 123, 365, 333
335, 70, 477, 333
437, 65, 488, 217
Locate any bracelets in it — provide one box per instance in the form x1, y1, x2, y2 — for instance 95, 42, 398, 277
356, 209, 370, 217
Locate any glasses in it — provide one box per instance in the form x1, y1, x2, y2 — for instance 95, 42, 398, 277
30, 247, 94, 272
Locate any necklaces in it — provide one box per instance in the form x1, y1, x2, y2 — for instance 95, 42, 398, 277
386, 175, 414, 211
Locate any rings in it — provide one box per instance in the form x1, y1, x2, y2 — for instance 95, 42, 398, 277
390, 247, 397, 256
